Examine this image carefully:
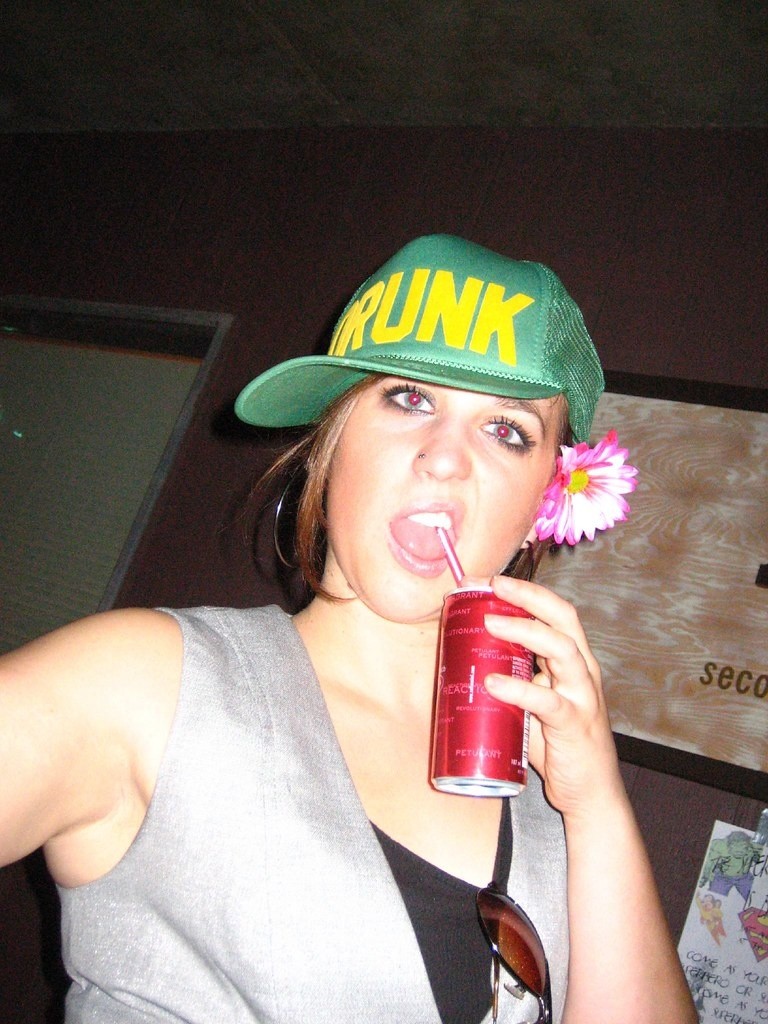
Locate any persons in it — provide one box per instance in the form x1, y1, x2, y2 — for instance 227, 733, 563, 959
1, 235, 702, 1023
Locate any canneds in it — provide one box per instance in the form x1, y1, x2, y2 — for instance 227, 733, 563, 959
432, 586, 530, 797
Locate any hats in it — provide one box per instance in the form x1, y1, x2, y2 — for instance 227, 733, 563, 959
233, 232, 606, 445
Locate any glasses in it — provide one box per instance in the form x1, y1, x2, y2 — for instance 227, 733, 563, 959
476, 884, 553, 1024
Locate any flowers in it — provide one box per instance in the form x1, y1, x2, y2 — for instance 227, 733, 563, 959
533, 428, 639, 546
